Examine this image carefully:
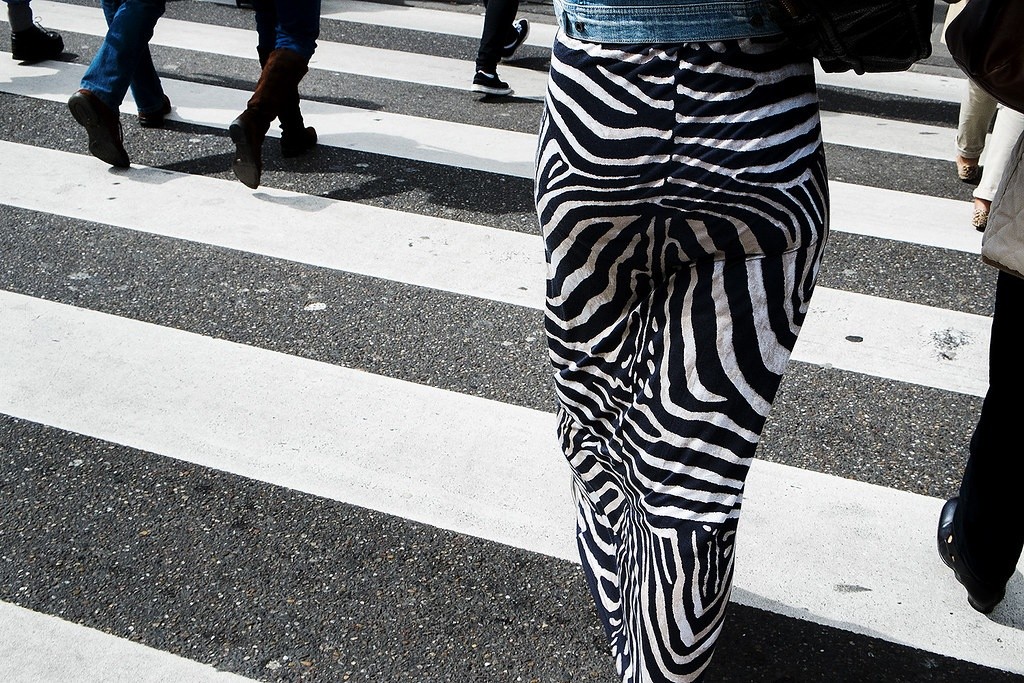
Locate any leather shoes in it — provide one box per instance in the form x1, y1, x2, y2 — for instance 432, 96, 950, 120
937, 496, 1000, 612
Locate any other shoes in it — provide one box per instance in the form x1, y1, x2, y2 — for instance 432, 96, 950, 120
955, 159, 978, 182
972, 208, 990, 229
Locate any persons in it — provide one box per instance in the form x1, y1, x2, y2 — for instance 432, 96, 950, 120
68, 0, 171, 168
228, 0, 321, 190
922, 0, 1024, 615
534, 0, 936, 683
470, 0, 528, 96
2, 0, 63, 62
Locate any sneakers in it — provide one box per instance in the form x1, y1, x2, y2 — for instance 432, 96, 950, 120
471, 70, 512, 95
139, 92, 172, 129
10, 15, 65, 61
68, 88, 130, 170
499, 18, 529, 60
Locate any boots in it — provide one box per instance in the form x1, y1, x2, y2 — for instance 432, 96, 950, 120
256, 42, 318, 160
228, 47, 310, 190
